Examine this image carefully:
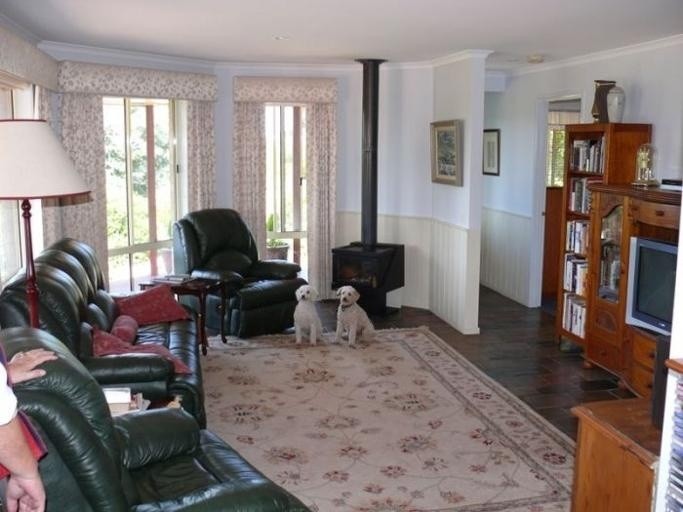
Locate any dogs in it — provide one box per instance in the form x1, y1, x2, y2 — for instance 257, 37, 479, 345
331, 285, 377, 349
293, 284, 322, 346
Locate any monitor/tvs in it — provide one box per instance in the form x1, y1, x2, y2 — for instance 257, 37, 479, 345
624, 236, 678, 337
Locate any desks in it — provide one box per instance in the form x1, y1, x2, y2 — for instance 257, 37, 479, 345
570, 398, 662, 512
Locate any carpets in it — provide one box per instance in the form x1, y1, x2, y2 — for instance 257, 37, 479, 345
199, 325, 577, 512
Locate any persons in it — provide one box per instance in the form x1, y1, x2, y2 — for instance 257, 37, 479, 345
0, 348, 58, 512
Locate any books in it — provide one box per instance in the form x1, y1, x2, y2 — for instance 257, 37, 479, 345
562, 136, 606, 339
102, 388, 151, 418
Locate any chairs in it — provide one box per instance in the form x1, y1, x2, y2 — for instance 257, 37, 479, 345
173, 209, 309, 339
0, 326, 310, 512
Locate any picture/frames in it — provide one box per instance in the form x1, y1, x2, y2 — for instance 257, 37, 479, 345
483, 129, 500, 175
430, 120, 464, 186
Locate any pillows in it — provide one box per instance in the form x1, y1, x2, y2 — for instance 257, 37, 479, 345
111, 315, 138, 344
117, 284, 192, 325
92, 329, 193, 375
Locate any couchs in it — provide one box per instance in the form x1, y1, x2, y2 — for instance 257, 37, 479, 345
0, 238, 206, 429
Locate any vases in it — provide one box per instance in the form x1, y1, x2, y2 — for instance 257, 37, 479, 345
607, 87, 625, 123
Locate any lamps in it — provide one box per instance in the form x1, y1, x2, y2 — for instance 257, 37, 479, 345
0, 120, 91, 328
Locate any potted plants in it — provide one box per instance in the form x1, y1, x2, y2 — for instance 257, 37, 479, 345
266, 214, 289, 260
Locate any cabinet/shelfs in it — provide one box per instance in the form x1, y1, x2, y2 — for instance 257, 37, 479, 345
556, 123, 650, 345
579, 191, 680, 399
